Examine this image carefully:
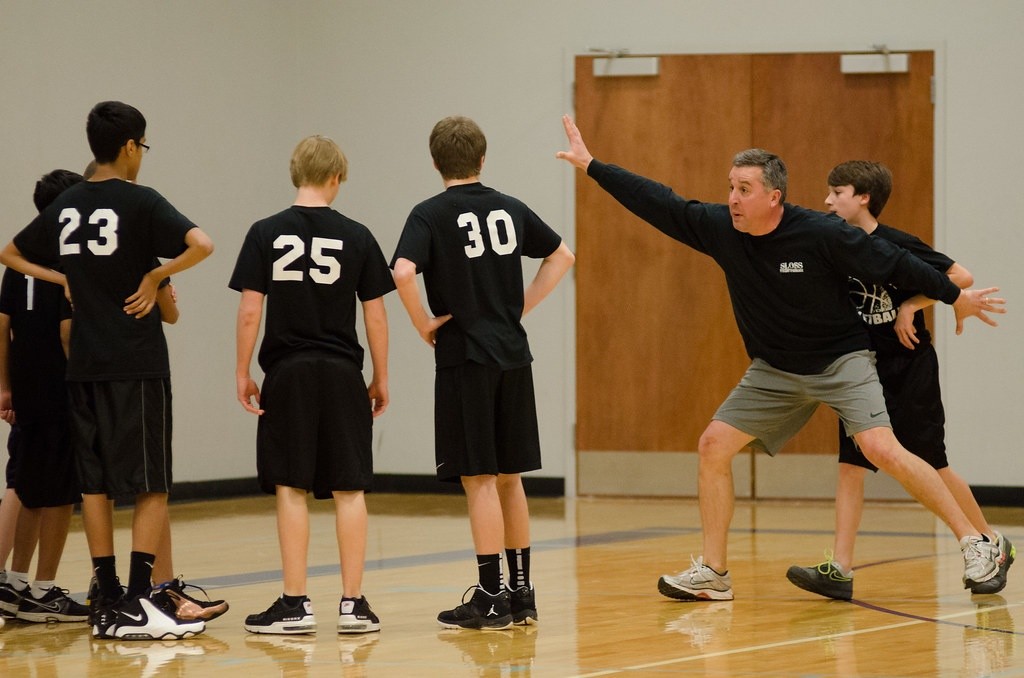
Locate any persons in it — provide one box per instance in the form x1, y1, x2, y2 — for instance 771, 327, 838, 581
0, 620, 534, 678
388, 114, 578, 630
228, 134, 399, 636
784, 157, 1015, 600
1, 102, 215, 641
556, 114, 1009, 601
658, 599, 1016, 678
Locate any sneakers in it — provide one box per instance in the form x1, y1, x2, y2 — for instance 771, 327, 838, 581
245, 633, 314, 667
505, 577, 537, 626
436, 583, 513, 629
658, 550, 734, 601
971, 531, 1016, 594
244, 594, 316, 635
88, 575, 125, 639
962, 532, 1003, 588
438, 627, 514, 665
786, 565, 853, 599
339, 636, 380, 667
91, 635, 228, 678
660, 601, 734, 643
115, 589, 207, 641
511, 624, 538, 659
143, 579, 229, 624
16, 589, 89, 622
337, 595, 380, 633
0, 581, 34, 620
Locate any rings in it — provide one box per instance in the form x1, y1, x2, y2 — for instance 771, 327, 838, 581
985, 298, 988, 305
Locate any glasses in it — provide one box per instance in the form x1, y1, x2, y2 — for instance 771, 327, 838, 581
122, 141, 151, 154
